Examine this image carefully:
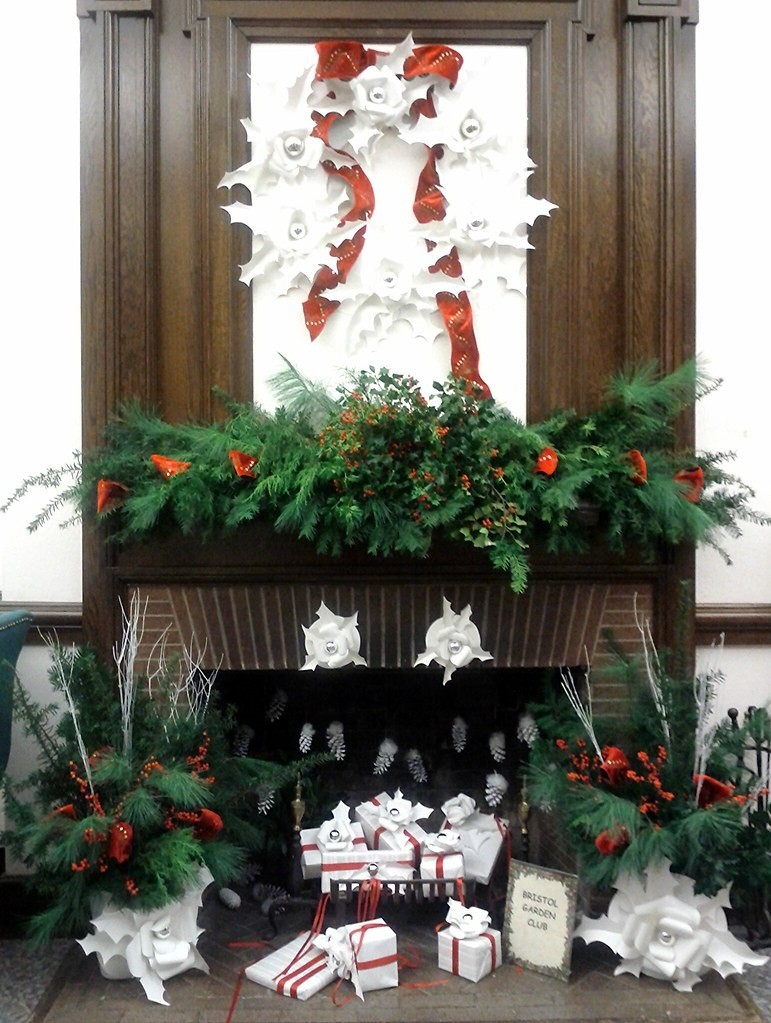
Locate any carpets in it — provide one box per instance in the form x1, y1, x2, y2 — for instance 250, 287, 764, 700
29, 946, 764, 1023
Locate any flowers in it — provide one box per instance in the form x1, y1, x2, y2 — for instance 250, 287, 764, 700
298, 600, 368, 671
0, 584, 290, 957
413, 595, 494, 686
215, 30, 561, 352
0, 349, 771, 568
522, 590, 771, 894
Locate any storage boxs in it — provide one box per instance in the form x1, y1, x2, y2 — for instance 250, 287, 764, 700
244, 789, 509, 1001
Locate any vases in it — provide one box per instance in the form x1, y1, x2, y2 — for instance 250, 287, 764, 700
572, 855, 770, 993
103, 512, 700, 582
74, 864, 215, 1006
